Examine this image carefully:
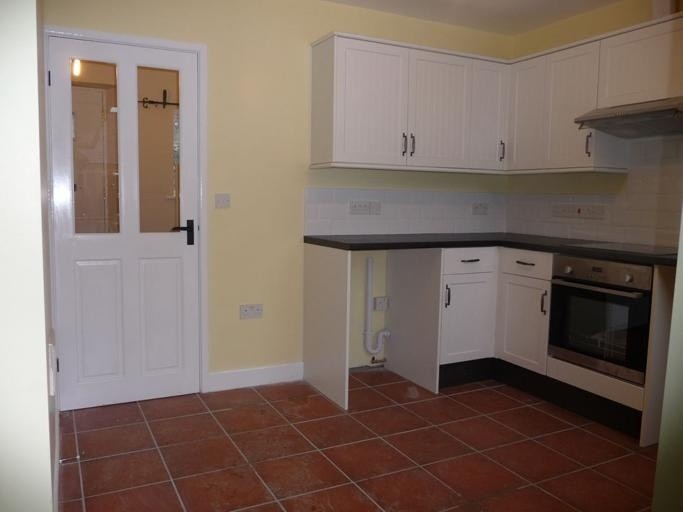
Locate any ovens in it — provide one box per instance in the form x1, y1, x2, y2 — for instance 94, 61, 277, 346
547, 277, 650, 373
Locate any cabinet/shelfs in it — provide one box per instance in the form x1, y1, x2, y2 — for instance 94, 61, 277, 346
494, 247, 555, 379
468, 52, 510, 174
1, 0, 63, 511
306, 30, 469, 174
439, 247, 498, 367
509, 35, 636, 173
598, 12, 682, 119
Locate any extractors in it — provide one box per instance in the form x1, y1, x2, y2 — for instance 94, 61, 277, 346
573, 96, 682, 141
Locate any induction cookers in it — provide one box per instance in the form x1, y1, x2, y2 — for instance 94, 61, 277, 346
553, 237, 677, 289
